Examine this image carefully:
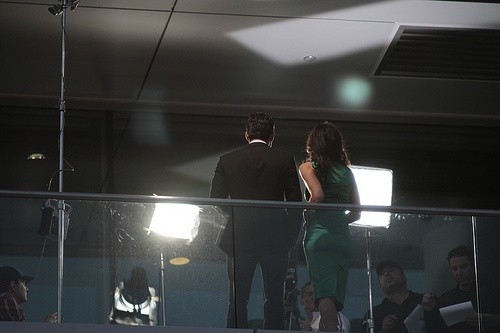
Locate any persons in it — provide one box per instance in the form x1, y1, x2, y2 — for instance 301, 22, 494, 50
422, 246, 500, 333
210, 109, 303, 330
299, 122, 361, 333
108, 265, 158, 325
0, 265, 65, 323
298, 281, 351, 333
362, 260, 425, 333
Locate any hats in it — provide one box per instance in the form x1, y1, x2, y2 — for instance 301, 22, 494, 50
375, 259, 402, 277
0, 266, 35, 285
